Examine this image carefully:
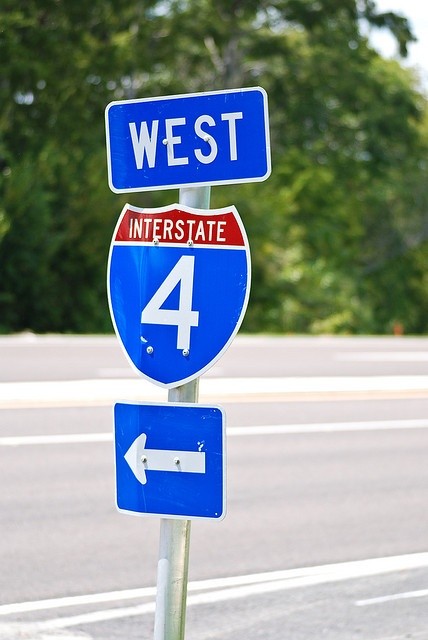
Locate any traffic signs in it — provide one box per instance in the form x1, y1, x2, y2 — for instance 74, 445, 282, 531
104, 86, 273, 194
107, 203, 252, 389
